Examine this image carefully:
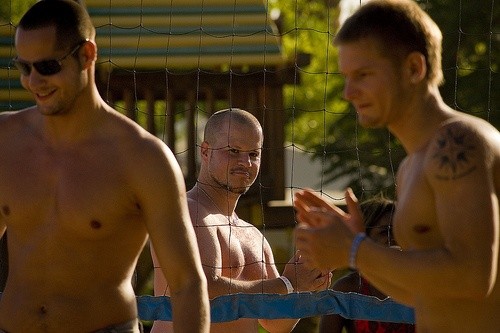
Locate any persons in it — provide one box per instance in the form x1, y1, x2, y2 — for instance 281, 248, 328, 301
147, 108, 333, 333
318, 197, 415, 333
293, 0, 500, 333
0, 0, 212, 333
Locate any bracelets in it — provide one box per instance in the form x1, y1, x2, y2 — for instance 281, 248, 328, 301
347, 232, 367, 267
277, 275, 295, 294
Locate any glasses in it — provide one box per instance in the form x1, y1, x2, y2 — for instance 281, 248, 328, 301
13, 38, 89, 76
